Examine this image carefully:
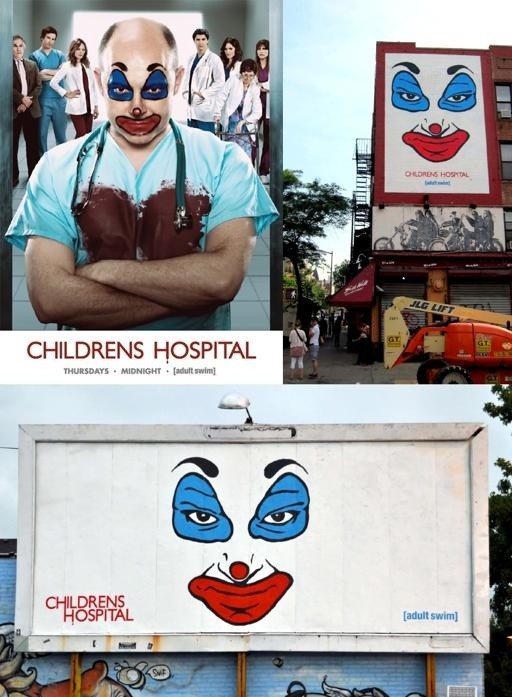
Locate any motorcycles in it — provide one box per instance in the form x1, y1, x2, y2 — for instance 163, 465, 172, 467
374, 221, 503, 251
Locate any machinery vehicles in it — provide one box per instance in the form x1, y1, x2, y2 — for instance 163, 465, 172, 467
382, 296, 511, 382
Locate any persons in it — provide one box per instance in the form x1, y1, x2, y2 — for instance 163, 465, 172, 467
289, 320, 308, 380
319, 316, 342, 347
352, 322, 370, 342
13, 26, 270, 188
3, 19, 280, 331
401, 210, 494, 251
308, 316, 320, 379
